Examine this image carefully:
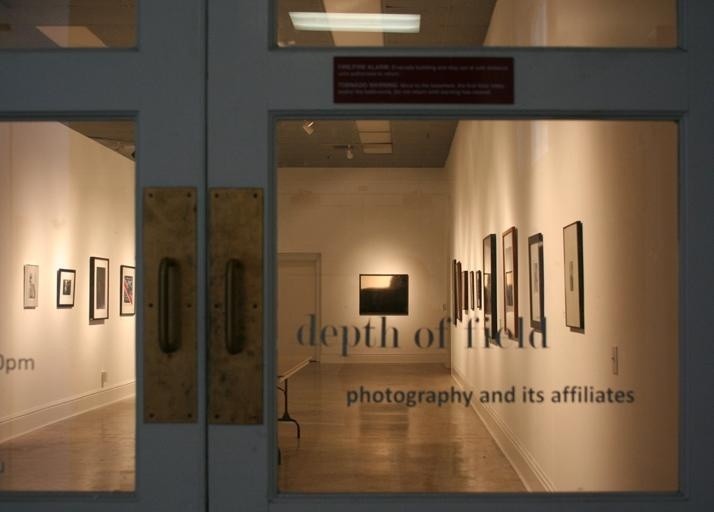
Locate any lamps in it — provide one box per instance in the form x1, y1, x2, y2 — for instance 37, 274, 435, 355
300, 120, 316, 138
341, 144, 353, 160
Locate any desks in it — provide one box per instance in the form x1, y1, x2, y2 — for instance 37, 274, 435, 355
279, 353, 314, 446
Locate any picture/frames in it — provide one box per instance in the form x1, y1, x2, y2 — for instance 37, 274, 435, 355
500, 226, 518, 339
525, 231, 545, 331
358, 273, 409, 317
560, 220, 585, 331
18, 256, 136, 322
480, 232, 497, 340
449, 258, 482, 326
